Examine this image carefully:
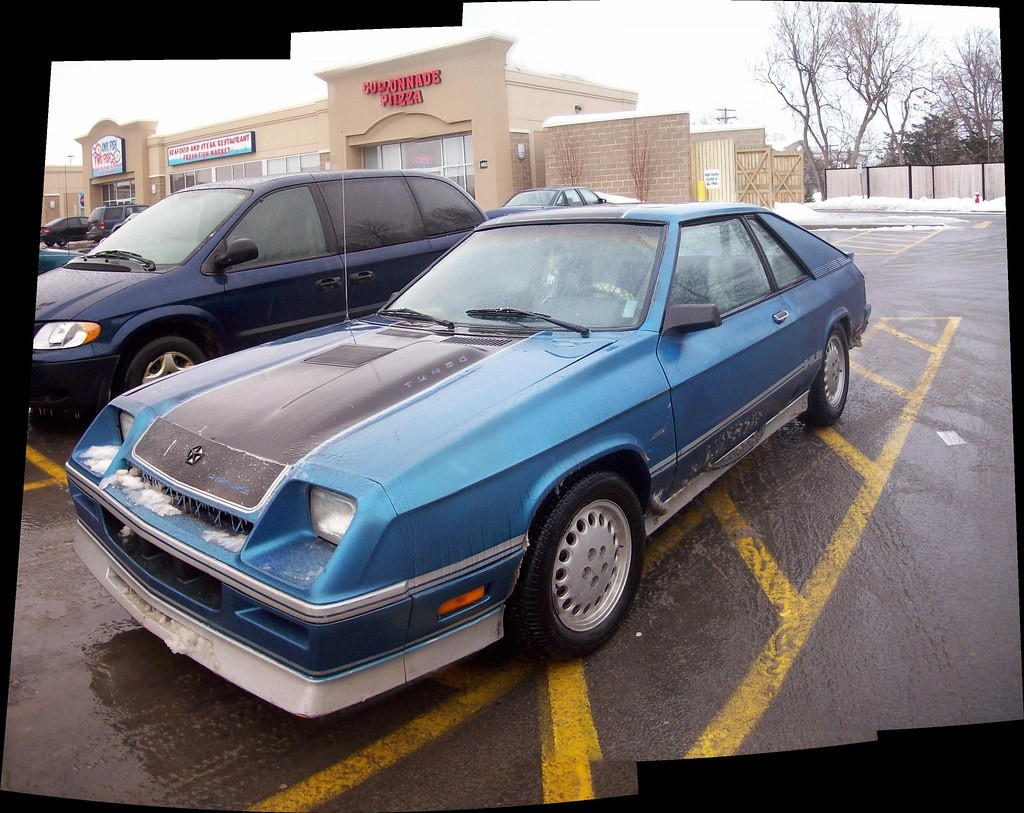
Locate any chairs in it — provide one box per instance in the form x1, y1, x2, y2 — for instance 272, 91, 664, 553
618, 244, 654, 299
556, 250, 596, 299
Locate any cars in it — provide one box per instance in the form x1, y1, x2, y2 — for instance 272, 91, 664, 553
86, 204, 150, 243
38, 245, 85, 274
486, 186, 610, 219
64, 203, 871, 720
31, 169, 489, 409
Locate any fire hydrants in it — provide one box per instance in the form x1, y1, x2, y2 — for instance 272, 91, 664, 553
975, 194, 980, 203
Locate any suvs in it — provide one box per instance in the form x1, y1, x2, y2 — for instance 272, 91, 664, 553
40, 216, 89, 246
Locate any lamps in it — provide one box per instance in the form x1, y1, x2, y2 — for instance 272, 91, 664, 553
478, 160, 488, 170
575, 105, 582, 113
